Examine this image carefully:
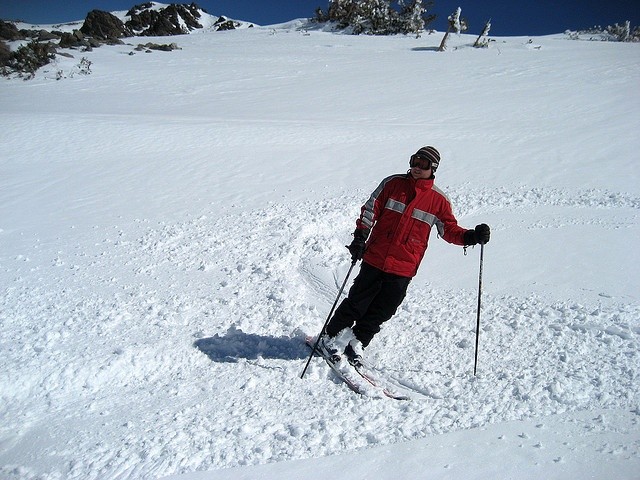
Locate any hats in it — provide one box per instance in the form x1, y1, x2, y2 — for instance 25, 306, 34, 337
415, 146, 441, 172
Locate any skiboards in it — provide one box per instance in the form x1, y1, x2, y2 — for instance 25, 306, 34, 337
305, 336, 411, 401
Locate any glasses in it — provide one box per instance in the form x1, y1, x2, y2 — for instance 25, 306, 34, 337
409, 155, 438, 171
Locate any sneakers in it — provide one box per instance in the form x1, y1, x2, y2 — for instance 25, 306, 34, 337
314, 336, 342, 365
345, 342, 365, 370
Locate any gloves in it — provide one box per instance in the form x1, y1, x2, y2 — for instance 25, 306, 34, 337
462, 223, 490, 246
348, 228, 370, 260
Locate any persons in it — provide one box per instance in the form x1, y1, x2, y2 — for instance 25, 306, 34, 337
315, 145, 490, 367
